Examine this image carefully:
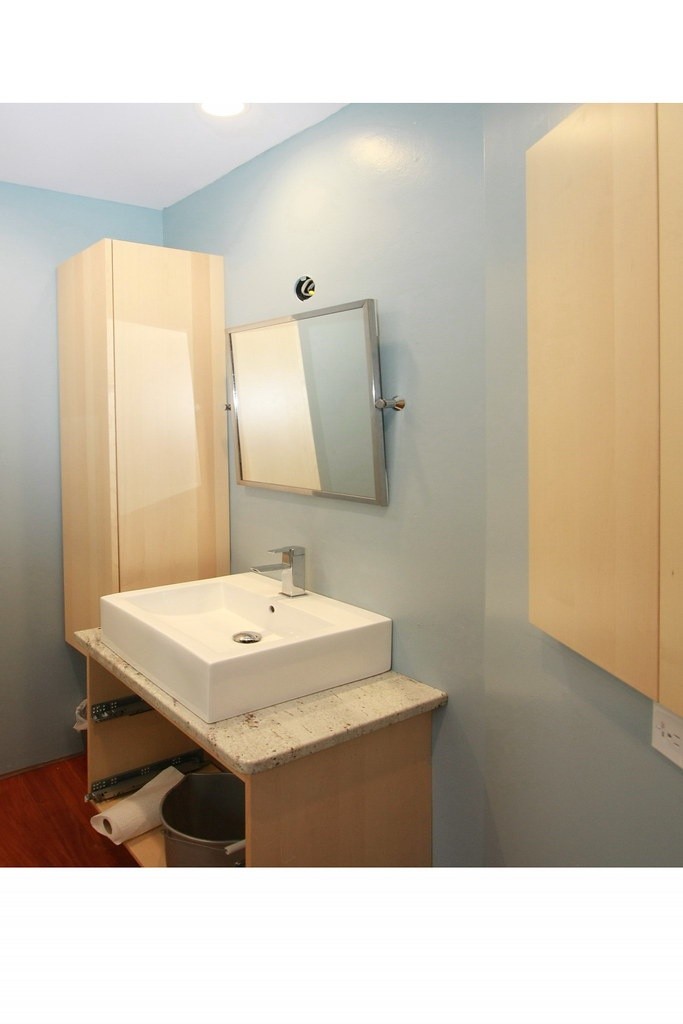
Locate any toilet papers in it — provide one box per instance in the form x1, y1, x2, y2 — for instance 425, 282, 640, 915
90, 766, 187, 845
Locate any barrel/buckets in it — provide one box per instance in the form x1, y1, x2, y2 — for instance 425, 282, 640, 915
159, 773, 245, 867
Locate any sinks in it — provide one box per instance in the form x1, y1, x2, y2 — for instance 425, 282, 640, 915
101, 572, 393, 725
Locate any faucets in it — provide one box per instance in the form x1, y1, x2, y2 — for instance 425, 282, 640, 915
250, 545, 306, 596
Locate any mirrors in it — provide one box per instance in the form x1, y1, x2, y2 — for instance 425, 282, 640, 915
224, 298, 389, 508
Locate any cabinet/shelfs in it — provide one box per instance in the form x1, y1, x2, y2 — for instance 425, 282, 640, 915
524, 104, 683, 721
87, 655, 444, 867
57, 238, 230, 656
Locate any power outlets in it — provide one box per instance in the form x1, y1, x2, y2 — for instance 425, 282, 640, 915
650, 702, 683, 769
